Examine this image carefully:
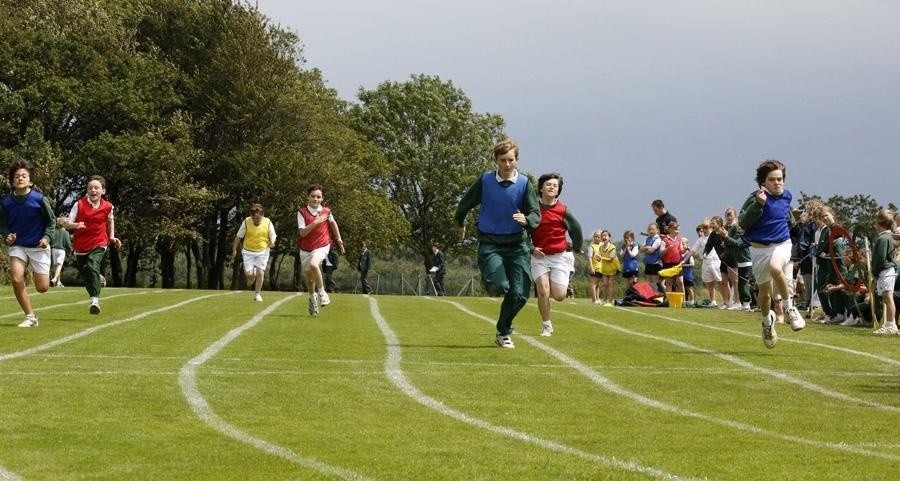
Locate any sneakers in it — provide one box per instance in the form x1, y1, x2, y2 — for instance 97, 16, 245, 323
50, 278, 57, 287
253, 294, 263, 302
541, 323, 554, 337
719, 303, 732, 310
872, 326, 898, 335
89, 300, 101, 315
307, 296, 320, 317
819, 315, 831, 323
727, 303, 740, 310
100, 274, 106, 288
317, 291, 331, 307
784, 307, 806, 330
17, 319, 39, 328
830, 314, 843, 323
495, 331, 515, 349
840, 316, 858, 326
740, 302, 750, 310
707, 300, 717, 307
56, 282, 65, 288
761, 310, 777, 350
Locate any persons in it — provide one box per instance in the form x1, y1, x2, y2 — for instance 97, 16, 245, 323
50, 217, 73, 288
588, 200, 900, 332
297, 186, 346, 316
322, 244, 339, 293
64, 176, 121, 314
431, 243, 446, 296
232, 202, 278, 302
738, 160, 807, 347
566, 243, 577, 298
526, 173, 584, 337
455, 143, 542, 349
357, 242, 374, 294
0, 160, 56, 328
